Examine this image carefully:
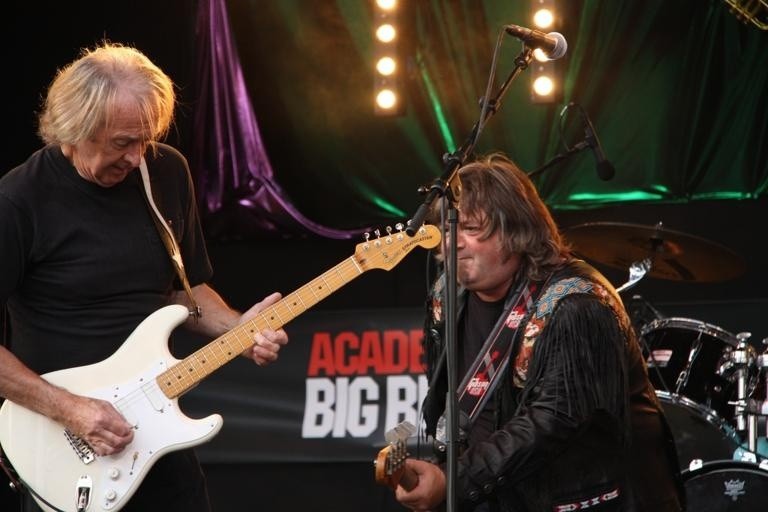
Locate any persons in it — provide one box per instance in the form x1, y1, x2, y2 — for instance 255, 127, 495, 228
393, 154, 689, 512
1, 43, 290, 512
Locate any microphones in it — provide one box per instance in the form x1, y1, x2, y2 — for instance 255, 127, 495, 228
504, 24, 569, 59
577, 101, 615, 183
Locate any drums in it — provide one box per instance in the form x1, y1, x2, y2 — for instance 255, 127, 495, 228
637, 316, 757, 460
750, 352, 768, 415
680, 454, 768, 511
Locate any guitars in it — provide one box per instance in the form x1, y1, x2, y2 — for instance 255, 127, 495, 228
374, 409, 468, 511
0, 221, 443, 512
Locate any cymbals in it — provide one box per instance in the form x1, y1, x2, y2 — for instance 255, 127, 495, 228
563, 220, 746, 283
